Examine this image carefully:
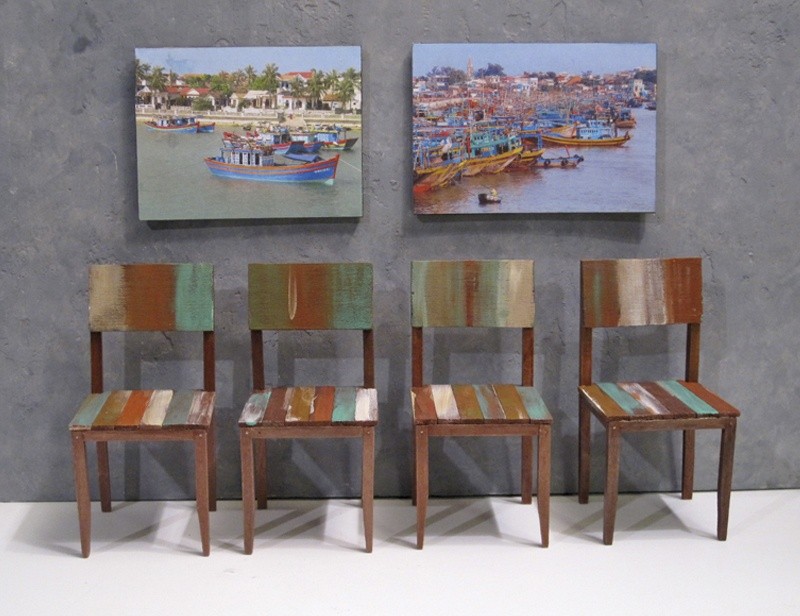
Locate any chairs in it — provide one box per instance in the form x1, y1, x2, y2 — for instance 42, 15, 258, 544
66, 262, 218, 559
237, 262, 379, 555
405, 259, 553, 551
579, 256, 742, 545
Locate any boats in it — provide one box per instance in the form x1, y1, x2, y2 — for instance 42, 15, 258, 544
143, 110, 360, 156
410, 56, 639, 195
202, 130, 341, 186
